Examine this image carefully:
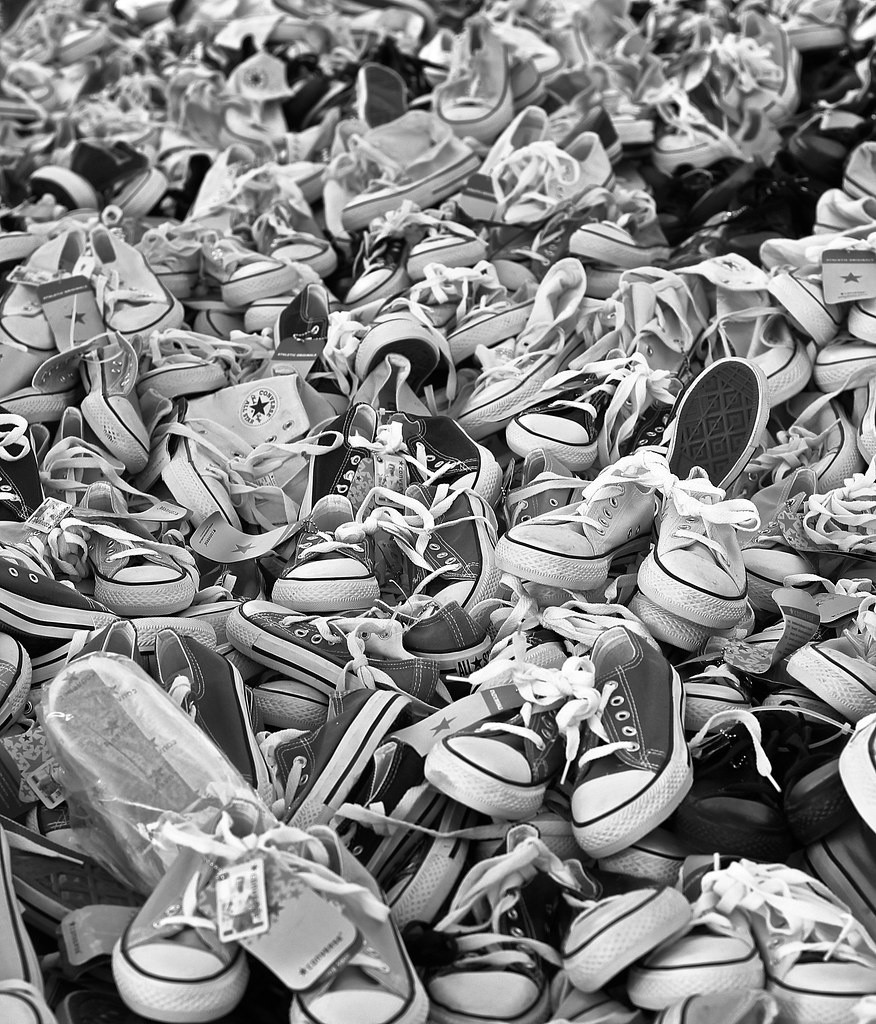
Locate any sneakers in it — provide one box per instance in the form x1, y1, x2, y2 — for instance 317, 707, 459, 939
0, 0, 875, 1023
